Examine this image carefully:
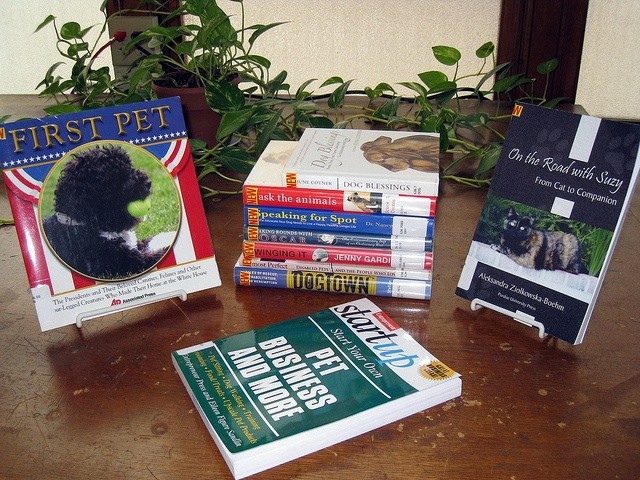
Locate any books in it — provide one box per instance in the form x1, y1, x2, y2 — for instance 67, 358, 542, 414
0, 96, 223, 333
242, 240, 434, 270
242, 140, 438, 219
281, 128, 440, 197
170, 298, 463, 480
240, 257, 433, 281
455, 101, 640, 345
233, 254, 432, 301
244, 205, 435, 239
243, 224, 434, 253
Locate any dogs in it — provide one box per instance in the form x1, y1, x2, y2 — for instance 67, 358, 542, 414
348, 193, 378, 213
42, 143, 170, 280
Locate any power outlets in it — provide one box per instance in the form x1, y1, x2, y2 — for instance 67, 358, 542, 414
107, 15, 162, 68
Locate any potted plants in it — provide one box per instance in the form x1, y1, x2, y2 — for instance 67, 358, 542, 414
0, 0, 569, 230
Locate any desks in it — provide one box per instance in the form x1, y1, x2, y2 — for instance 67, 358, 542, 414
0, 94, 640, 480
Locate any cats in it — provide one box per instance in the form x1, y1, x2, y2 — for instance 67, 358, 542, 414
490, 206, 589, 275
